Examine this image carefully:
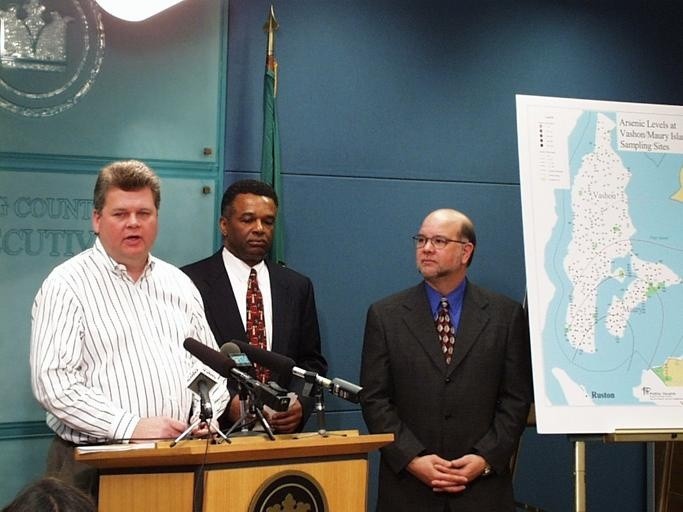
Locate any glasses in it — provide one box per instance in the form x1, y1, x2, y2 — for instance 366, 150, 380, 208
412, 234, 466, 249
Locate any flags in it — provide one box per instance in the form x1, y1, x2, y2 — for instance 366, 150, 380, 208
261, 70, 285, 267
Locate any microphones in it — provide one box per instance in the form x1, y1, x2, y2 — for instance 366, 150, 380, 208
181, 357, 222, 418
183, 334, 291, 414
228, 333, 367, 405
214, 342, 262, 403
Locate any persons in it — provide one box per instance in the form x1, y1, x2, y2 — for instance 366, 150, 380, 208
179, 180, 329, 434
358, 207, 533, 511
0, 477, 96, 512
28, 158, 231, 478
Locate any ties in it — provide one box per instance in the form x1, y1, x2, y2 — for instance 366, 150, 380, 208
243, 269, 271, 431
434, 297, 456, 365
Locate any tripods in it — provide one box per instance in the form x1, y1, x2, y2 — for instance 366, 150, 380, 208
169, 401, 232, 449
292, 387, 350, 440
218, 397, 280, 444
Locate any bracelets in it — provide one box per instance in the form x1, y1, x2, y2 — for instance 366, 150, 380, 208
482, 462, 491, 476
